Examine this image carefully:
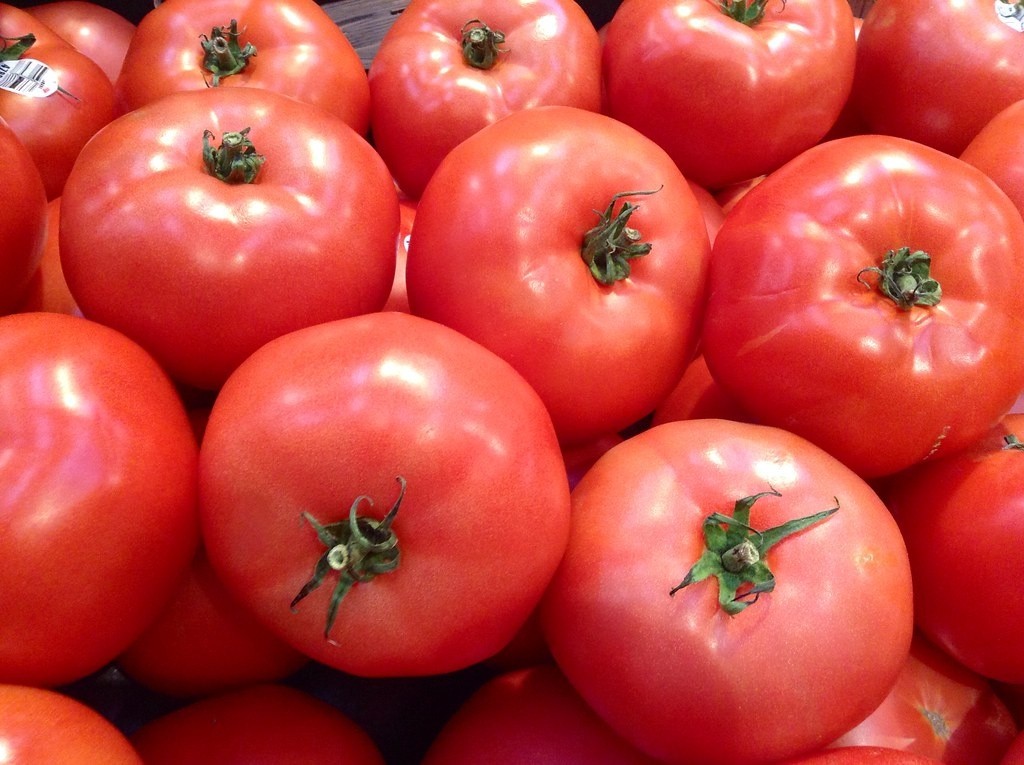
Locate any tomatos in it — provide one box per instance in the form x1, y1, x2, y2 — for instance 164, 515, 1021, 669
0, 1, 1024, 764
